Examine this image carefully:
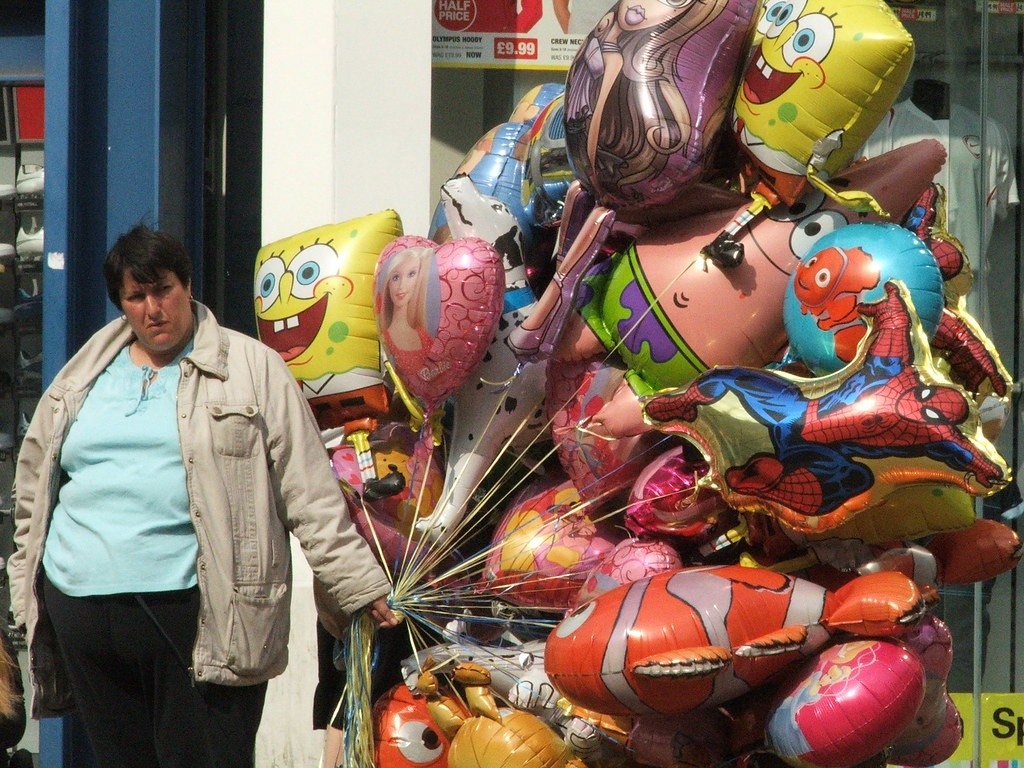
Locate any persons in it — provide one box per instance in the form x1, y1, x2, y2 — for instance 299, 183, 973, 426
6, 223, 405, 768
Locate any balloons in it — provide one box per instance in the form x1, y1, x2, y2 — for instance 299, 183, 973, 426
253, 0, 1024, 768
